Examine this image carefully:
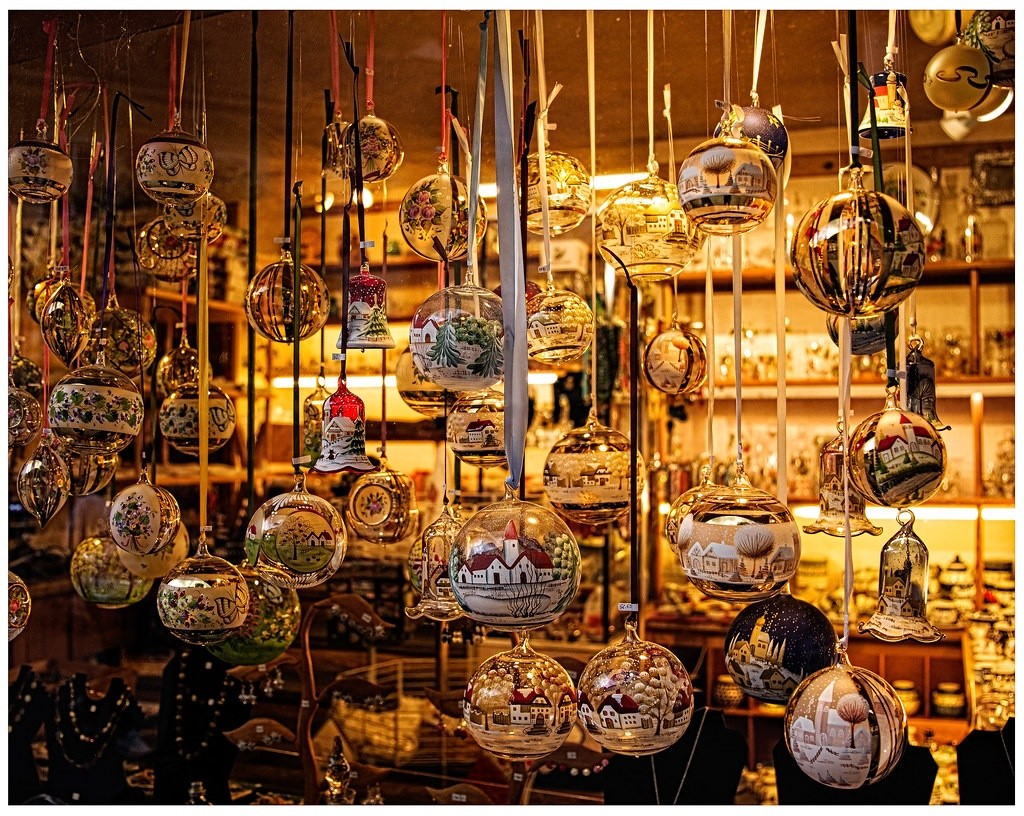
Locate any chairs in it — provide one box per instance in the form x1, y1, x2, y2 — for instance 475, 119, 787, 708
328, 559, 407, 646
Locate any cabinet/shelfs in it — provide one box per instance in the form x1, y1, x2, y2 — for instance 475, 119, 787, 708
51, 117, 1013, 731
